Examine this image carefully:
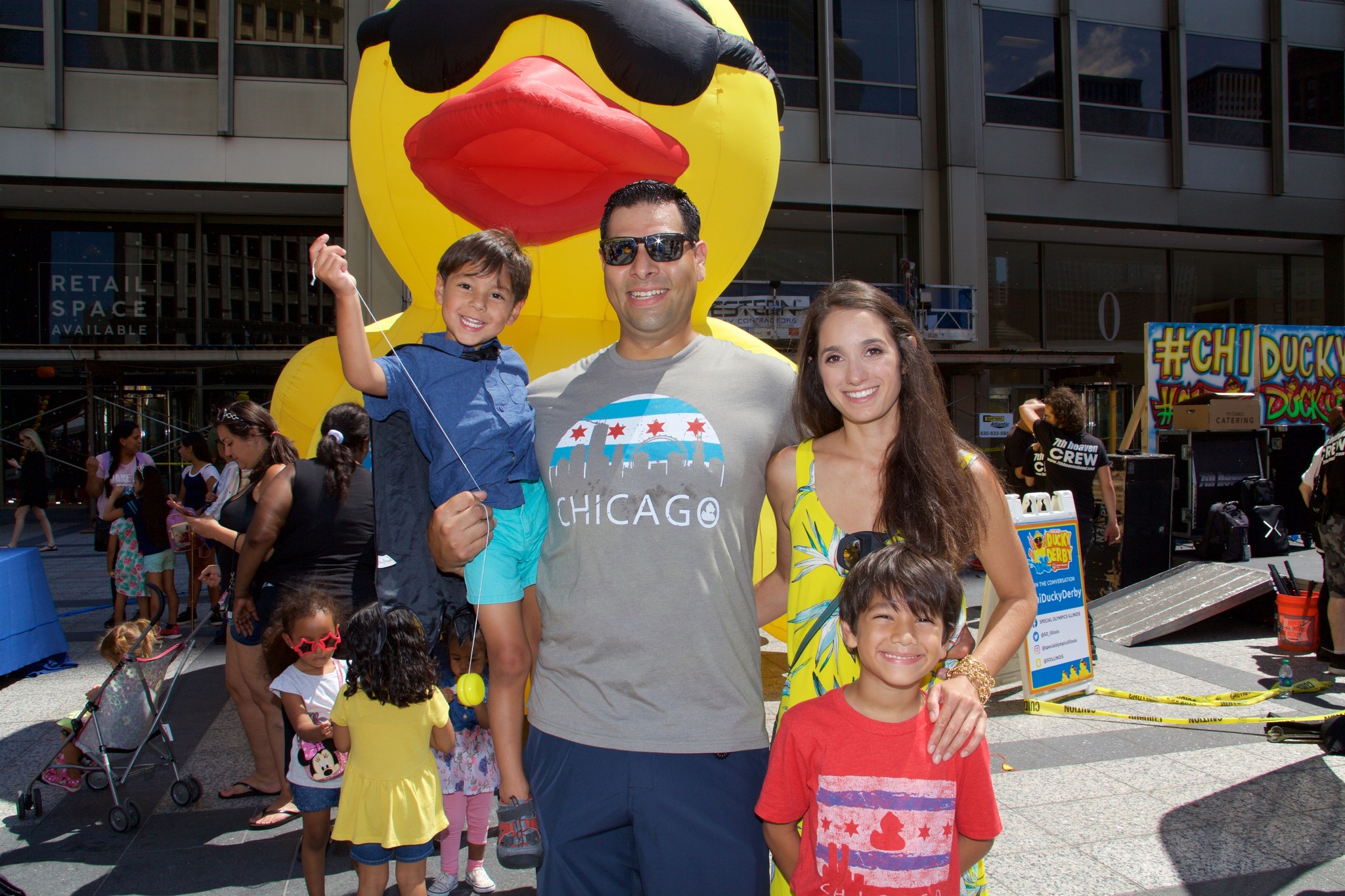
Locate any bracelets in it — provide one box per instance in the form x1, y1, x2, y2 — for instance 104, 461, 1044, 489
110, 569, 115, 576
946, 654, 996, 704
17, 465, 22, 470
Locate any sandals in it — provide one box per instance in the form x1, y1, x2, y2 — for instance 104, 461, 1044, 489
39, 544, 58, 551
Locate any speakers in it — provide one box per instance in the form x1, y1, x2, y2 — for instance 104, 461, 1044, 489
1086, 450, 1177, 599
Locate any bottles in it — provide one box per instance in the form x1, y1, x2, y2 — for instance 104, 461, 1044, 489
122, 485, 134, 495
1108, 587, 1113, 594
1278, 660, 1292, 697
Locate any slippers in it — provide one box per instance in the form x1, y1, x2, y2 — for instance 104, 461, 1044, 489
250, 807, 302, 831
219, 781, 281, 799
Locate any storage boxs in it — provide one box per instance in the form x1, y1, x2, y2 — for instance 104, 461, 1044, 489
1155, 429, 1270, 540
1172, 392, 1261, 431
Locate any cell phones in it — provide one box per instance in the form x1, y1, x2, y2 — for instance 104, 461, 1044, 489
167, 500, 195, 518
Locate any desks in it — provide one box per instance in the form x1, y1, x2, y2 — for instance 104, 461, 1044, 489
0, 547, 79, 678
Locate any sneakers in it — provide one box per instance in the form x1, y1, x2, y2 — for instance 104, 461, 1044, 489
427, 873, 458, 896
465, 867, 495, 893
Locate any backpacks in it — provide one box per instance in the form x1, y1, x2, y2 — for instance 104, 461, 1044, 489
1251, 505, 1290, 555
1235, 475, 1273, 517
1208, 501, 1249, 560
166, 506, 205, 552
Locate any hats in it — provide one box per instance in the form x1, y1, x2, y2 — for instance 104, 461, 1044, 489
1327, 403, 1345, 436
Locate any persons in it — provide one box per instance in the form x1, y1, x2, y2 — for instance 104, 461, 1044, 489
85, 420, 157, 629
197, 437, 257, 645
755, 542, 1003, 896
1018, 386, 1120, 661
41, 616, 162, 795
102, 464, 182, 640
330, 601, 456, 896
0, 428, 58, 552
167, 432, 224, 626
427, 178, 975, 896
207, 394, 235, 476
107, 492, 151, 626
180, 400, 303, 831
751, 274, 1037, 895
263, 587, 360, 896
1298, 398, 1345, 676
232, 402, 376, 864
427, 602, 500, 896
1003, 398, 1048, 512
308, 229, 551, 870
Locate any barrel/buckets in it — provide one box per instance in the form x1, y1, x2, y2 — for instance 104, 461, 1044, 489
1276, 590, 1320, 652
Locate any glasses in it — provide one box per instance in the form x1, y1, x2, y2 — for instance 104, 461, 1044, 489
599, 232, 688, 267
289, 627, 341, 656
18, 439, 25, 443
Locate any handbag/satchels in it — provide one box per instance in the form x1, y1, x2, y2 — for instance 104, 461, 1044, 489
94, 517, 110, 552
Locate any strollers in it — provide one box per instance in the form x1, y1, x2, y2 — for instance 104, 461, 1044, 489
13, 582, 233, 834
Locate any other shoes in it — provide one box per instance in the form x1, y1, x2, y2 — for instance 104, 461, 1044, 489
177, 607, 198, 626
159, 625, 183, 640
1327, 654, 1345, 674
210, 608, 224, 626
42, 769, 82, 794
497, 794, 544, 871
105, 613, 127, 629
